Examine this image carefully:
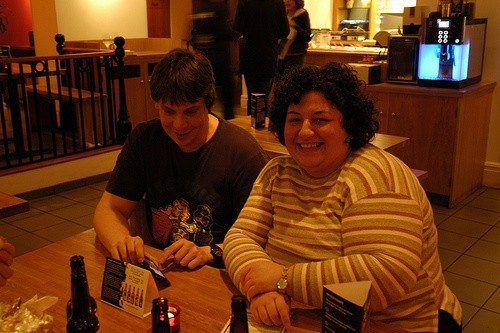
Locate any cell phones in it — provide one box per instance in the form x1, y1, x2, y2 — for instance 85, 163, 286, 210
143, 255, 170, 287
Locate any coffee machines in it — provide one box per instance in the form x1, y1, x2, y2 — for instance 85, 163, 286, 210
385, 0, 489, 89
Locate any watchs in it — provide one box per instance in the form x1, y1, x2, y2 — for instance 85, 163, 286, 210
276, 266, 289, 294
209, 244, 222, 265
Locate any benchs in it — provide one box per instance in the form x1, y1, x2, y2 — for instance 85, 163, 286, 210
23, 83, 108, 151
406, 166, 428, 181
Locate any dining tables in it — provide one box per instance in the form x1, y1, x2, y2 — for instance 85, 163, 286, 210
220, 114, 411, 164
0, 227, 410, 333
1, 43, 35, 57
0, 57, 67, 80
0, 188, 31, 219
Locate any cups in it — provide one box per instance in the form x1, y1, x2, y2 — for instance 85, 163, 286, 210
167, 304, 180, 333
320, 33, 331, 49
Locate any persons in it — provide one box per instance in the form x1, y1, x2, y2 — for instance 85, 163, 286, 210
93, 48, 267, 270
0, 237, 15, 290
221, 63, 464, 333
229, 0, 290, 114
263, 0, 312, 131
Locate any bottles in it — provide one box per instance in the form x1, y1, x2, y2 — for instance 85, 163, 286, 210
66, 255, 100, 333
151, 298, 171, 333
123, 284, 144, 308
229, 294, 249, 333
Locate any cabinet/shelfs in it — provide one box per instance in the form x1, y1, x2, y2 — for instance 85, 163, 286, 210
300, 47, 388, 71
330, 0, 380, 46
147, 0, 242, 105
351, 80, 496, 210
62, 33, 185, 148
